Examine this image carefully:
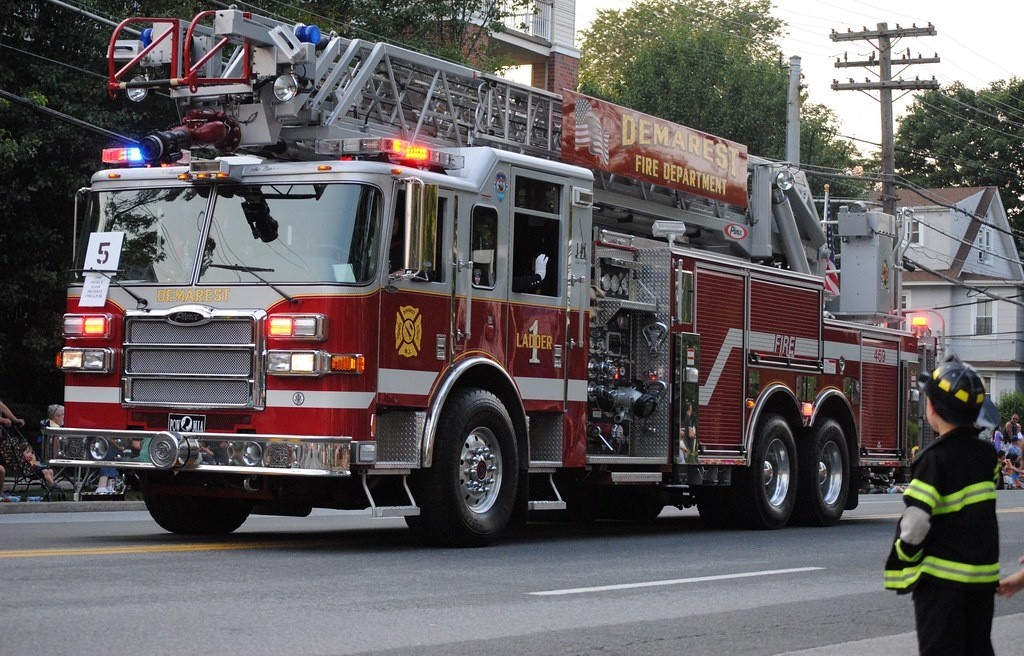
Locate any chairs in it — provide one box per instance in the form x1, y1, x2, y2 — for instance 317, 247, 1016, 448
37, 437, 127, 502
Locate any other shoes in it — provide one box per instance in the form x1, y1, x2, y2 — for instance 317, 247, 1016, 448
96, 487, 117, 492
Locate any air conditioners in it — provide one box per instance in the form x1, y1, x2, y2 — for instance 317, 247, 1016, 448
979, 252, 989, 261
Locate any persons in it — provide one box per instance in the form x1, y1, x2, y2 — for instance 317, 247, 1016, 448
0, 400, 118, 501
996, 555, 1024, 597
883, 356, 1000, 656
855, 413, 1024, 494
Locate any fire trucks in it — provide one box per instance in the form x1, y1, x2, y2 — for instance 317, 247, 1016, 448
42, 4, 945, 552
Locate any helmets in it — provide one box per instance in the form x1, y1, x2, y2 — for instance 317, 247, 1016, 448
923, 362, 986, 419
